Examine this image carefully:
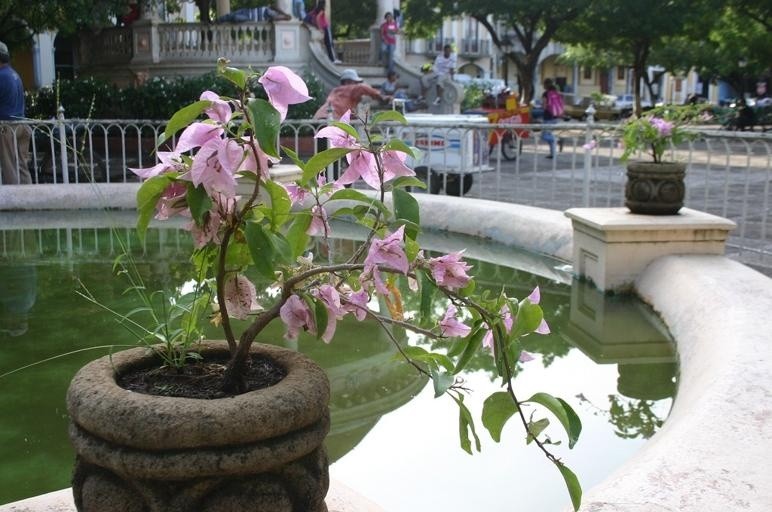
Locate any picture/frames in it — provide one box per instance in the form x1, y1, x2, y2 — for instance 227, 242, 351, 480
616, 363, 675, 400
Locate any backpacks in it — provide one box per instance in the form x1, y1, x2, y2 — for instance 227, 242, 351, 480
547, 89, 564, 117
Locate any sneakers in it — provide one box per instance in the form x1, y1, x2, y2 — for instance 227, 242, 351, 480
413, 95, 442, 107
332, 59, 342, 65
545, 155, 553, 159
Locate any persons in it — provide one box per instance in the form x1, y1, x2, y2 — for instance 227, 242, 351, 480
292, 0, 307, 20
0, 40, 33, 184
312, 69, 391, 181
304, 2, 343, 64
380, 13, 398, 70
734, 98, 758, 131
414, 44, 458, 106
541, 79, 564, 158
382, 71, 428, 111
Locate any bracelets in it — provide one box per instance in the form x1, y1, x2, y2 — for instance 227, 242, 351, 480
382, 39, 385, 42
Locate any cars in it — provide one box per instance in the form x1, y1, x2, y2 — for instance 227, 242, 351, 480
578, 93, 771, 123
450, 74, 543, 114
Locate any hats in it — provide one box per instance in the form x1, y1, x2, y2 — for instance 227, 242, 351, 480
340, 69, 363, 83
0, 40, 9, 56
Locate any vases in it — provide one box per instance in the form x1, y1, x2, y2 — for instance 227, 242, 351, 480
65, 336, 331, 512
625, 161, 686, 214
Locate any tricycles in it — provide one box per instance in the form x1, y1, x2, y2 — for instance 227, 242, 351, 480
340, 97, 496, 197
466, 104, 541, 161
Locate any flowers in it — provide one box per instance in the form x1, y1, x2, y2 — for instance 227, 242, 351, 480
582, 101, 738, 163
575, 392, 663, 440
127, 58, 584, 512
